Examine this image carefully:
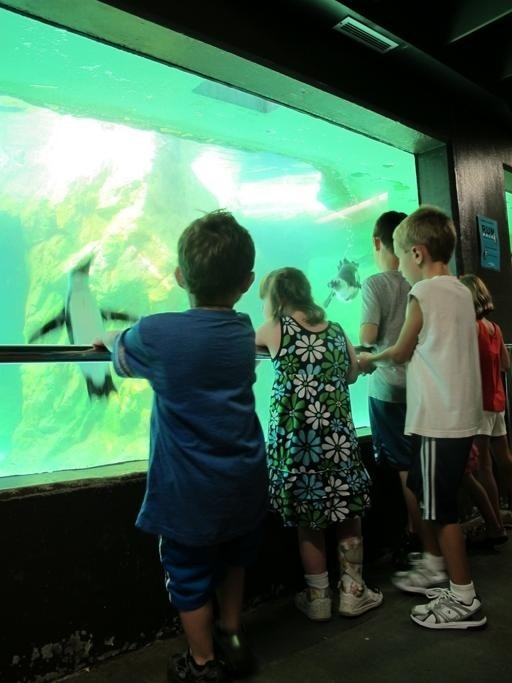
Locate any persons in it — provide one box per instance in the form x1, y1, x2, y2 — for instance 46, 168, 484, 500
255, 266, 386, 621
356, 205, 510, 632
90, 208, 274, 683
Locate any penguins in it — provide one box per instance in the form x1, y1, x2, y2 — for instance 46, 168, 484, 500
27, 251, 139, 407
322, 258, 361, 308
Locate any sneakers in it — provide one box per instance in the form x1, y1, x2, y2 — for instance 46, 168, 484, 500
212, 625, 256, 679
168, 649, 228, 683
338, 584, 384, 617
294, 590, 332, 622
409, 587, 487, 630
470, 526, 509, 548
391, 552, 450, 595
